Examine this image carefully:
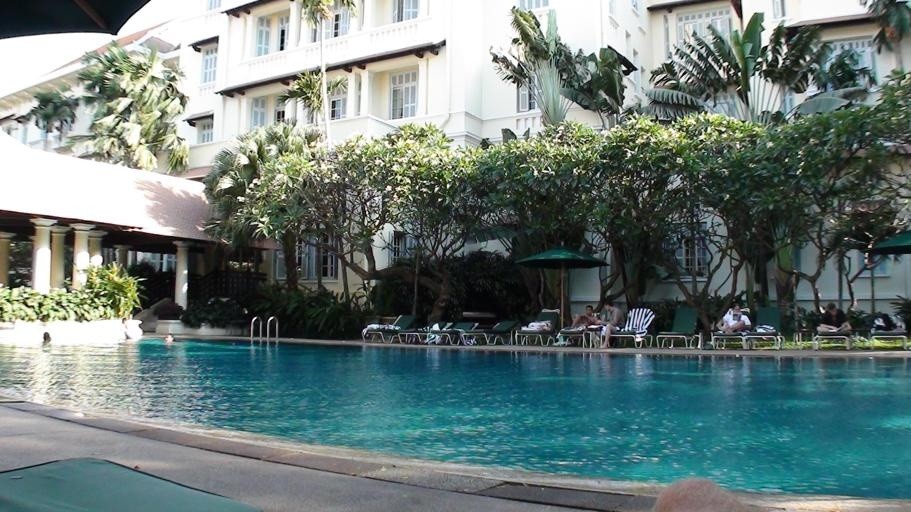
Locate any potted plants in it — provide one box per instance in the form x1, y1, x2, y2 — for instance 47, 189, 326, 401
0, 260, 262, 350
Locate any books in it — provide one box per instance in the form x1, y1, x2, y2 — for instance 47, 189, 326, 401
733, 314, 743, 320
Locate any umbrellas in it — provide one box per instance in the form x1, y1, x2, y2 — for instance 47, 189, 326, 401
871, 232, 911, 256
511, 246, 611, 342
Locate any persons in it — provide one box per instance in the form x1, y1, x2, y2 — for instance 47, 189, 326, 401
119, 317, 133, 341
571, 303, 600, 329
715, 302, 752, 335
599, 299, 625, 348
42, 331, 52, 341
870, 313, 905, 331
164, 332, 175, 345
816, 301, 854, 336
